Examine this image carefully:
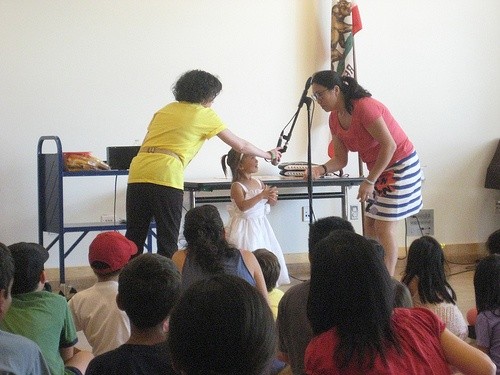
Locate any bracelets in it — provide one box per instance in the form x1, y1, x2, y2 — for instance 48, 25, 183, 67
321, 164, 327, 173
439, 243, 446, 252
263, 150, 275, 163
365, 178, 375, 185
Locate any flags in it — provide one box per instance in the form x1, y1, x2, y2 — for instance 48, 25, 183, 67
324, 1, 363, 159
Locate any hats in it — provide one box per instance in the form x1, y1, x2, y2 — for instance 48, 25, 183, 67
7, 243, 49, 284
89, 232, 138, 273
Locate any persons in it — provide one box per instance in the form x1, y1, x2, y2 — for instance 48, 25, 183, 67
473, 253, 500, 374
67, 231, 138, 355
303, 69, 423, 278
169, 270, 278, 375
302, 230, 497, 374
4, 240, 94, 374
221, 147, 292, 286
246, 247, 288, 372
83, 253, 181, 375
387, 276, 415, 310
124, 70, 285, 258
402, 235, 469, 340
1, 243, 50, 375
466, 227, 500, 327
170, 203, 269, 310
277, 214, 355, 375
368, 237, 385, 262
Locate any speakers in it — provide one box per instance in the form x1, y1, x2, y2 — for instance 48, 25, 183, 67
106, 146, 139, 169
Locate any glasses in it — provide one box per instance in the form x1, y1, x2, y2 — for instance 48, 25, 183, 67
311, 88, 329, 101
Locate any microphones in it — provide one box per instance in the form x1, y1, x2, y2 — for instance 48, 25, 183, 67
271, 131, 284, 165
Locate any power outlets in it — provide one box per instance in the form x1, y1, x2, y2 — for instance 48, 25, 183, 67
302, 206, 311, 222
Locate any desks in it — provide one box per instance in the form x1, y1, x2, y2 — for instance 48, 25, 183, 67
179, 175, 368, 239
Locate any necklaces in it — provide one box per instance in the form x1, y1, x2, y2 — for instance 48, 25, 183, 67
336, 111, 351, 130
